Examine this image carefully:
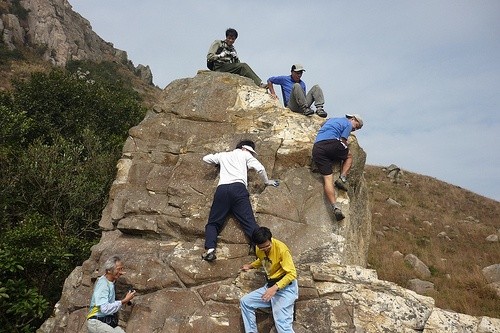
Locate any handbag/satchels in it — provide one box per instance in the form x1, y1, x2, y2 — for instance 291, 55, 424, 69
265, 273, 292, 291
89, 316, 118, 328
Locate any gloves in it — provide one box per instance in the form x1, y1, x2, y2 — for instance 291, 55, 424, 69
265, 179, 279, 187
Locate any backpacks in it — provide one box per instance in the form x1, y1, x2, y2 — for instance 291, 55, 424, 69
207, 41, 224, 71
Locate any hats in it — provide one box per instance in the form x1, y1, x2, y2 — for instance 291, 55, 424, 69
346, 114, 363, 129
291, 64, 305, 72
242, 145, 256, 154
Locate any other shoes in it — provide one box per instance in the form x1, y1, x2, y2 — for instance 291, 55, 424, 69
201, 252, 216, 261
333, 208, 345, 221
302, 108, 314, 116
316, 109, 327, 117
335, 178, 348, 191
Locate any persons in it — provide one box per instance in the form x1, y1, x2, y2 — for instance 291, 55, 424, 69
240, 227, 298, 333
310, 114, 364, 221
202, 139, 279, 260
206, 29, 269, 89
86, 256, 136, 333
267, 64, 328, 118
